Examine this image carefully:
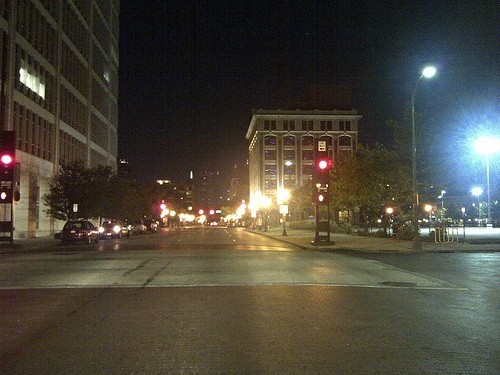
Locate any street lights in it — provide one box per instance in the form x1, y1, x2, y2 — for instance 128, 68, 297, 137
412, 66, 437, 250
278, 189, 289, 236
387, 207, 394, 236
480, 137, 494, 227
472, 186, 482, 227
263, 198, 271, 232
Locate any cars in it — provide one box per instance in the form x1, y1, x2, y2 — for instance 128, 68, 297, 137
98, 219, 159, 239
59, 220, 101, 245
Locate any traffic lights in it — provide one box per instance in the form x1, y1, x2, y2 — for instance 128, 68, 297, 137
317, 152, 330, 182
0, 131, 15, 181
